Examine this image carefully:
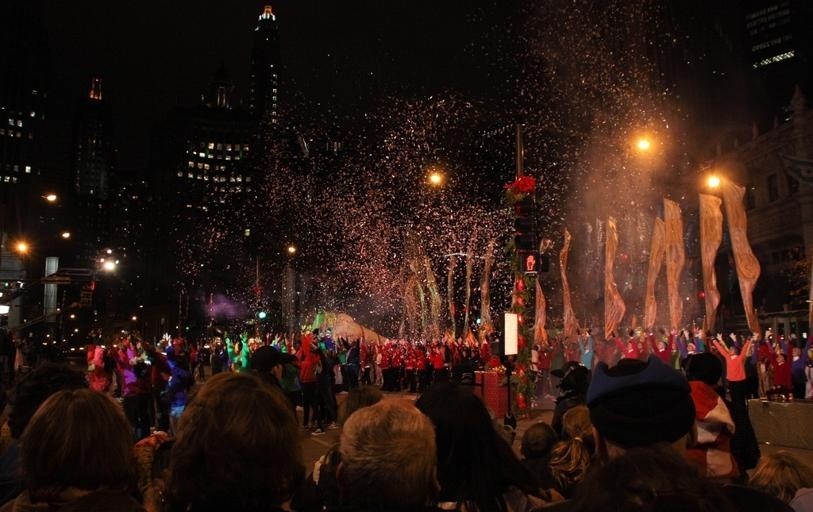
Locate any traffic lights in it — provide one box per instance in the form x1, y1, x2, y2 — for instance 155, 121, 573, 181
513, 193, 538, 250
255, 308, 269, 323
517, 250, 540, 273
